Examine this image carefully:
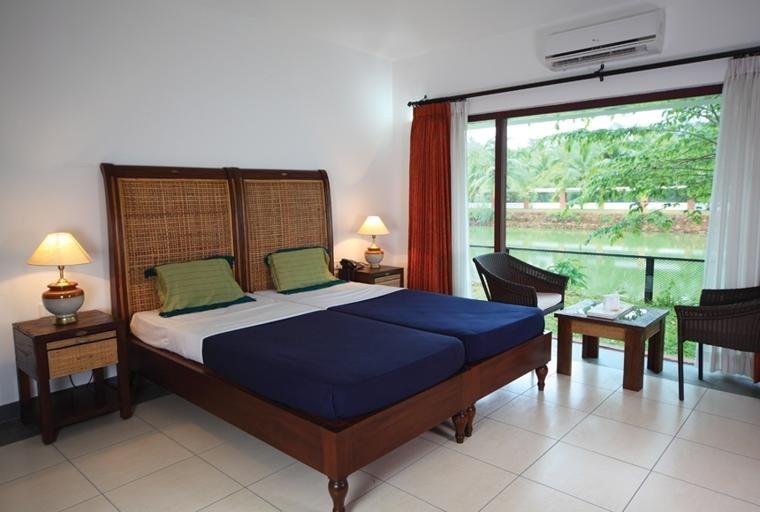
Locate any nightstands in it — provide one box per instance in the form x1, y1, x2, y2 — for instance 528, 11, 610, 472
338, 264, 404, 289
13, 310, 132, 444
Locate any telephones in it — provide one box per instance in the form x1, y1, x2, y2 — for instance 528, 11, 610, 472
340, 258, 363, 270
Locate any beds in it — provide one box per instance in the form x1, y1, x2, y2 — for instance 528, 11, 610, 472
99, 162, 552, 512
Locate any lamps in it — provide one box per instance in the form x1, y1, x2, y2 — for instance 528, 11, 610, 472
26, 232, 93, 327
358, 215, 390, 269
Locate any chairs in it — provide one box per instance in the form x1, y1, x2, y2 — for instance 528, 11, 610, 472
473, 252, 570, 317
673, 286, 760, 400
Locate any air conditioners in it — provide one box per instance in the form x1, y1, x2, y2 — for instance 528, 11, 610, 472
537, 8, 665, 71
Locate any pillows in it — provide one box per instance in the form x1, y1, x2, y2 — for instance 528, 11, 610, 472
145, 255, 257, 318
265, 247, 343, 295
